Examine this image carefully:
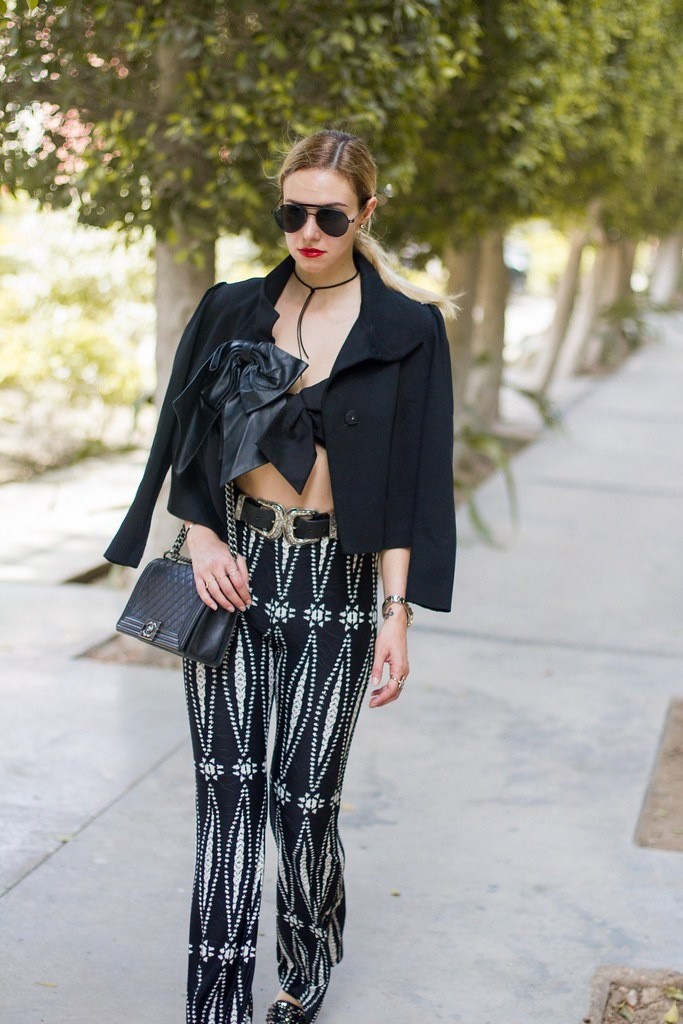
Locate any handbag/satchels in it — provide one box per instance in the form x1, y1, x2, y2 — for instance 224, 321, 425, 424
116, 560, 237, 668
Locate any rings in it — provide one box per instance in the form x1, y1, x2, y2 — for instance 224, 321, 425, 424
391, 671, 405, 690
227, 569, 239, 577
205, 578, 214, 588
216, 574, 226, 582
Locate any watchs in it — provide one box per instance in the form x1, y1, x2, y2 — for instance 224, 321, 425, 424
381, 594, 414, 629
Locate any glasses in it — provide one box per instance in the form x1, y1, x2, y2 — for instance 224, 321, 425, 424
270, 196, 368, 238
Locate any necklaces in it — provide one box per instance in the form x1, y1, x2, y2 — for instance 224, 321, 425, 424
293, 267, 361, 383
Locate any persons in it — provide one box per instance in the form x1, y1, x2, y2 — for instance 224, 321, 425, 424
107, 129, 461, 1024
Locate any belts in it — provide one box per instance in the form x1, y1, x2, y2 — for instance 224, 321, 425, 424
230, 490, 339, 546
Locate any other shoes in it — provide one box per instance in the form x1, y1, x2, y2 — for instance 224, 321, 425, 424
265, 1000, 307, 1024
